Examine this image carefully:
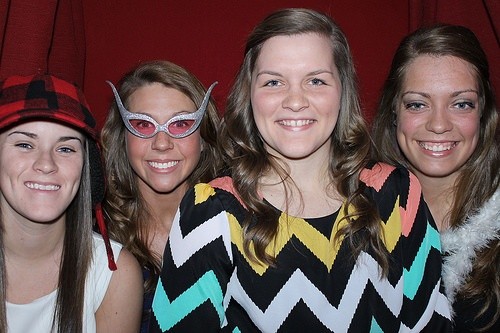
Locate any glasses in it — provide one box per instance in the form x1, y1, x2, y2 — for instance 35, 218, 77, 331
106, 81, 218, 139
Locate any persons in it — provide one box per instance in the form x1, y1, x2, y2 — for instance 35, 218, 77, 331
145, 8, 453, 333
98, 60, 219, 333
369, 21, 500, 333
0, 67, 145, 333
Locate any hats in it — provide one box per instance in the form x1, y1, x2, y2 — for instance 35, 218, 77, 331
0, 73, 99, 141
